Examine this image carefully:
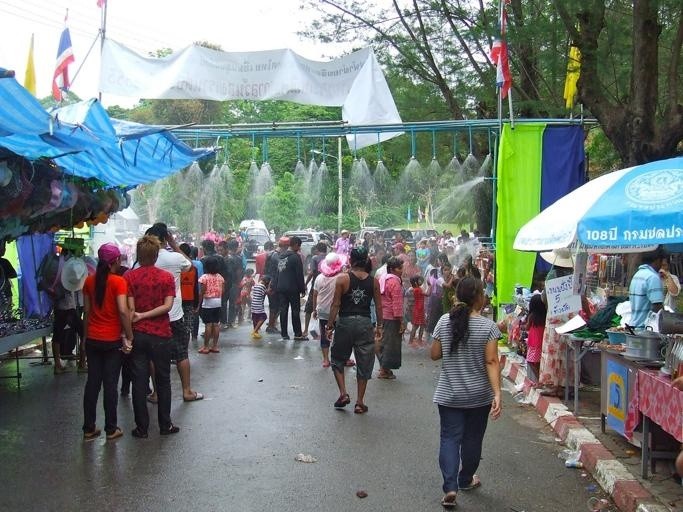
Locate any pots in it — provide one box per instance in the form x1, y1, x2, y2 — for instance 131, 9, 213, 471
624, 325, 665, 361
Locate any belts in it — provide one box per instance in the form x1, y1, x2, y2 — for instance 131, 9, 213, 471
340, 314, 364, 319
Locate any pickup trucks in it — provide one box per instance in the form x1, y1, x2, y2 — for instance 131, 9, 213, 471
254, 228, 335, 276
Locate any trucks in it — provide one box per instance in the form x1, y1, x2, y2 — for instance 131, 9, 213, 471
238, 219, 271, 262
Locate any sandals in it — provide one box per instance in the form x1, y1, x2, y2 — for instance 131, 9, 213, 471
283, 336, 290, 340
539, 385, 574, 400
199, 317, 272, 337
322, 361, 330, 368
333, 393, 350, 407
354, 403, 368, 413
344, 359, 355, 366
197, 346, 210, 354
376, 369, 396, 379
293, 333, 309, 341
209, 347, 219, 353
528, 382, 543, 388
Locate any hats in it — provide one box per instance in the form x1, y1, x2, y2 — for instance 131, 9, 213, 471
98, 242, 122, 263
539, 248, 574, 268
317, 249, 348, 278
57, 238, 86, 251
0, 153, 133, 242
341, 226, 482, 259
60, 256, 89, 291
180, 223, 250, 258
278, 236, 290, 245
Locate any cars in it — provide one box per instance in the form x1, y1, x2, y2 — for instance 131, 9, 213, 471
347, 227, 440, 255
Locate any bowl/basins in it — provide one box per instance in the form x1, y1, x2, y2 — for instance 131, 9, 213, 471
605, 329, 625, 346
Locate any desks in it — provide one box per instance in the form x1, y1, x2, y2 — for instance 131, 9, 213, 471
557, 333, 602, 417
597, 347, 657, 473
1, 315, 60, 380
623, 371, 682, 479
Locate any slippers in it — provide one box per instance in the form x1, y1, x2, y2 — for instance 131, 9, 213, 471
146, 394, 160, 404
439, 496, 457, 506
458, 474, 481, 490
183, 392, 204, 402
406, 335, 439, 350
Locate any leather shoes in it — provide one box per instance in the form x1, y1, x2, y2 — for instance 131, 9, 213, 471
131, 427, 148, 438
265, 326, 281, 334
119, 387, 152, 395
159, 423, 179, 435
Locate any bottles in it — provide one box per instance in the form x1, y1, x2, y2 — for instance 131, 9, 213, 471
564, 460, 583, 468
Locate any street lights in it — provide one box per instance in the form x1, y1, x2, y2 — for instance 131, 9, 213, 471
309, 148, 342, 237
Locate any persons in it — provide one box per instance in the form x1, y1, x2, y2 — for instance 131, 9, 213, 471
430, 277, 502, 506
0, 239, 17, 306
53, 223, 682, 440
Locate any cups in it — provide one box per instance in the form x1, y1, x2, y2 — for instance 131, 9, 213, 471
587, 496, 602, 512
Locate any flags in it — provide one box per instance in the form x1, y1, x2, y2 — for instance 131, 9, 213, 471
25, 40, 37, 96
52, 14, 75, 103
490, 1, 510, 99
564, 23, 584, 109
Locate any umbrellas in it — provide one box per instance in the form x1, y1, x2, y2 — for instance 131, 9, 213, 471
512, 156, 683, 254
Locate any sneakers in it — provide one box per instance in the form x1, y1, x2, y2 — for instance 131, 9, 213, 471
83, 430, 101, 442
54, 366, 73, 374
106, 426, 124, 439
77, 366, 88, 372
